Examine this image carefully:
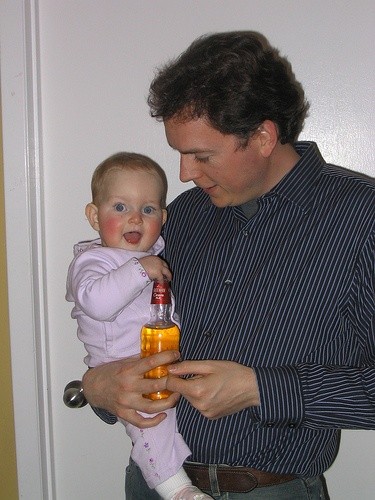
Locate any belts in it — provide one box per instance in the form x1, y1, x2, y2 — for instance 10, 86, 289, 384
183, 464, 296, 492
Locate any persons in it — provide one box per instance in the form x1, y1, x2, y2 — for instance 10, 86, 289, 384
82, 27, 375, 500
63, 151, 215, 500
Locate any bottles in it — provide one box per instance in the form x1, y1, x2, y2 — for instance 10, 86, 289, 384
140, 276, 179, 409
